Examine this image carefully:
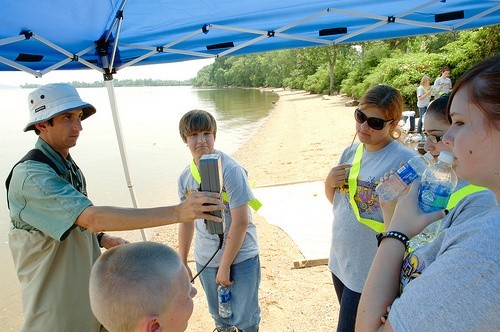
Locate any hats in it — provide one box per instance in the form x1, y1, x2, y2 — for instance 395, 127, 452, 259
21, 82, 97, 132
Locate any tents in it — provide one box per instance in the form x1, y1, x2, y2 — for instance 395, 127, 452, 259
0, 0, 500, 244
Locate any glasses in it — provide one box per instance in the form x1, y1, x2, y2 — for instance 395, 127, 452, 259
353, 106, 394, 131
421, 130, 445, 144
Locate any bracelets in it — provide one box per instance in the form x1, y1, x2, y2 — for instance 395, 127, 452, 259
97, 232, 105, 248
376, 231, 410, 250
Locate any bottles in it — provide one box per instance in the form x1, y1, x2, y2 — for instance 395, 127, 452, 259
375, 152, 434, 203
416, 150, 458, 243
217, 284, 233, 318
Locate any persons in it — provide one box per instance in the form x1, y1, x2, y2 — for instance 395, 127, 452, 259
417, 76, 431, 134
355, 55, 500, 332
325, 85, 424, 331
177, 110, 261, 331
434, 68, 452, 98
89, 242, 197, 332
379, 95, 496, 241
5, 83, 226, 332
